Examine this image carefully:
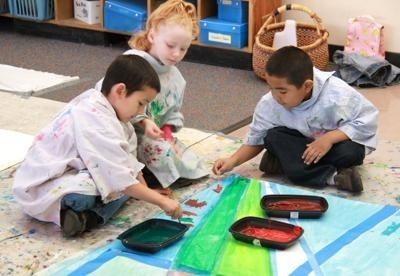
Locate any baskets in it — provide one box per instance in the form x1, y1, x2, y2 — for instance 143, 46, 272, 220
253, 4, 329, 82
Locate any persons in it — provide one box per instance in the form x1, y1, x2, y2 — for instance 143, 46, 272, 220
212, 45, 381, 193
93, 0, 211, 188
10, 54, 184, 237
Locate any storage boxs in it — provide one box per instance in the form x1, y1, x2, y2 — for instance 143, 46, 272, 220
198, 0, 248, 49
74, 0, 147, 34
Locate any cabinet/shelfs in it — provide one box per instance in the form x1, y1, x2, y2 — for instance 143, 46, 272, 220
0, 0, 285, 71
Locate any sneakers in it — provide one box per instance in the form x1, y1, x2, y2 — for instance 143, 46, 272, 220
259, 150, 282, 174
61, 207, 88, 237
334, 167, 363, 192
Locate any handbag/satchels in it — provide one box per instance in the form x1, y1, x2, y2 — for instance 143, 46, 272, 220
344, 15, 386, 59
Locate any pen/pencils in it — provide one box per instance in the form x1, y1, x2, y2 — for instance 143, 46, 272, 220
178, 218, 181, 223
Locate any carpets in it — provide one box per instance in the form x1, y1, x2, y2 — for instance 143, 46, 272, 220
0, 28, 343, 138
